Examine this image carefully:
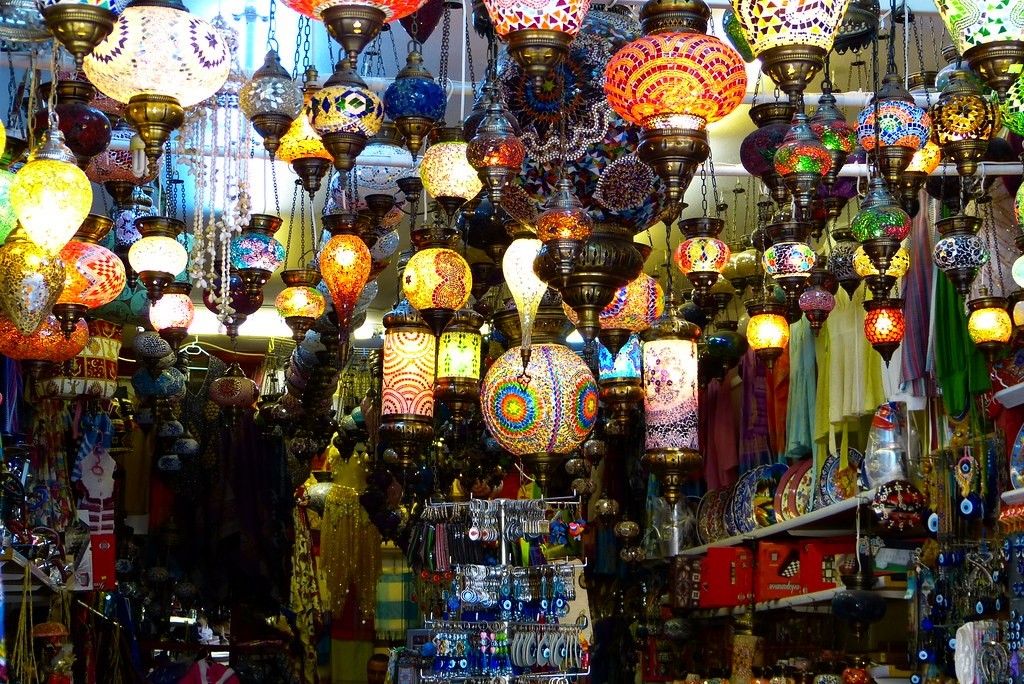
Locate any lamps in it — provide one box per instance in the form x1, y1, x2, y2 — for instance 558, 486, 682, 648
0, 0, 1024, 496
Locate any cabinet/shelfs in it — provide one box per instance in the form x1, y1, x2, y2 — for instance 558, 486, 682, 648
680, 382, 1024, 684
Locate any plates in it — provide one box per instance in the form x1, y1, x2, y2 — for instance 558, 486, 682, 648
1009, 422, 1024, 489
686, 448, 869, 548
